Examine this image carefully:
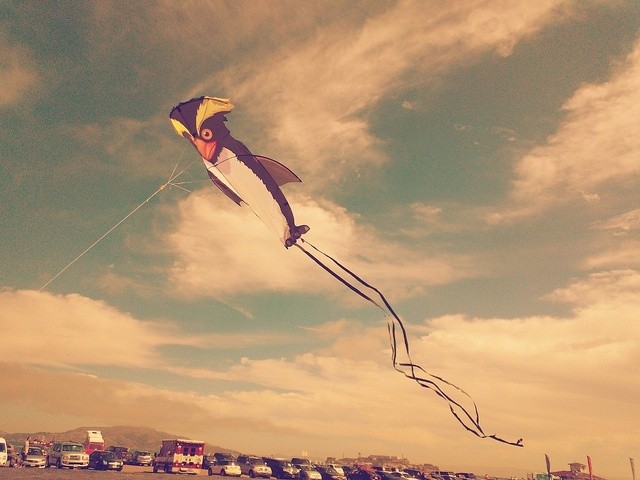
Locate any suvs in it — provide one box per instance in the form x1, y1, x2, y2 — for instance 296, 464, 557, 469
266, 459, 299, 479
437, 471, 456, 480
315, 466, 347, 480
133, 450, 151, 465
46, 442, 89, 469
238, 454, 272, 478
455, 473, 476, 480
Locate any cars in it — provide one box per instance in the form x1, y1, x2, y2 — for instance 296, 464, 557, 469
208, 460, 241, 477
18, 447, 46, 468
380, 471, 418, 480
346, 470, 380, 480
88, 450, 123, 471
294, 464, 322, 480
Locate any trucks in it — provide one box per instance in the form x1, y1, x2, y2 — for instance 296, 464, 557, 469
154, 439, 205, 473
83, 431, 104, 455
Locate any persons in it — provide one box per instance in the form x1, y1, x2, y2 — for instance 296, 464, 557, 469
19, 436, 32, 468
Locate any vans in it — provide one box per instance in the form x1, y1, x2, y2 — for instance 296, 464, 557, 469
0, 438, 8, 466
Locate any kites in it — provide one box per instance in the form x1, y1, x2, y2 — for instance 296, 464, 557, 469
168, 96, 523, 449
